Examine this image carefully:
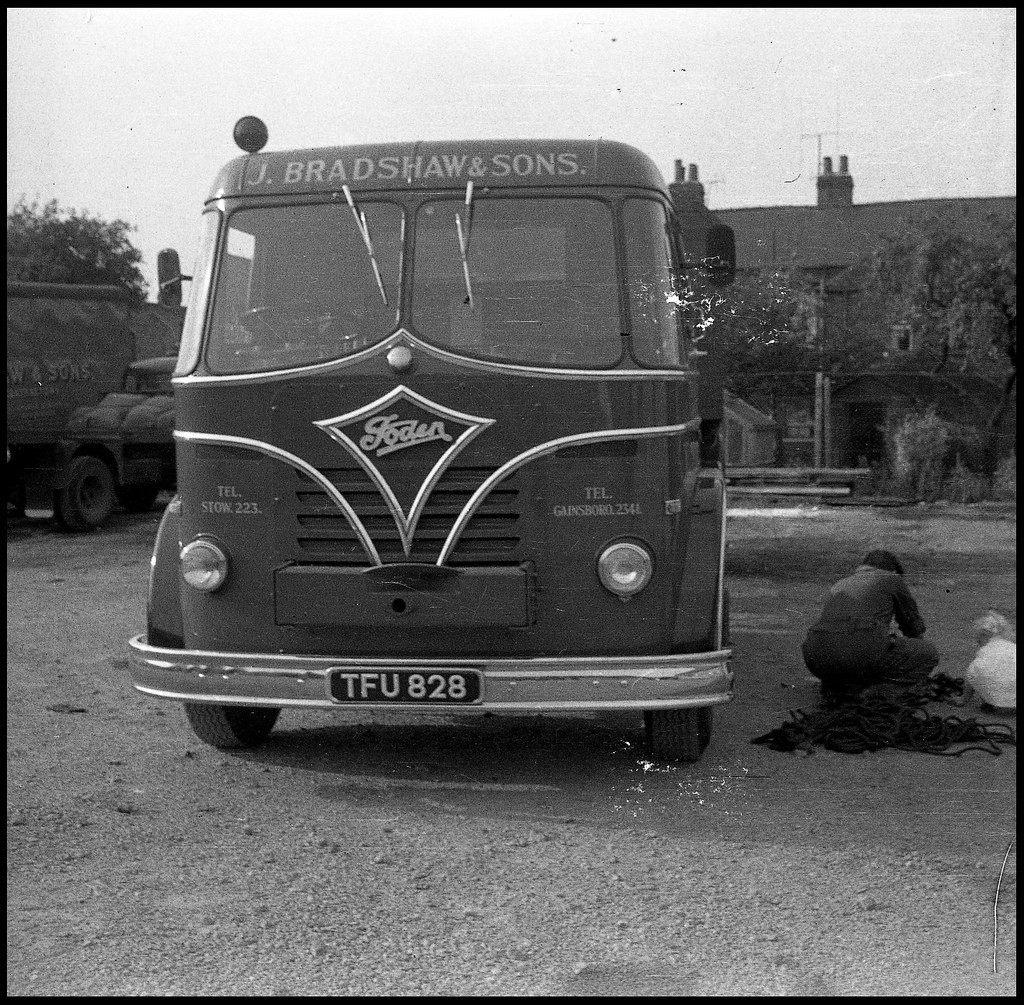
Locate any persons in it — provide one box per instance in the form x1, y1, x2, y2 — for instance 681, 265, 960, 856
953, 612, 1016, 716
802, 549, 940, 707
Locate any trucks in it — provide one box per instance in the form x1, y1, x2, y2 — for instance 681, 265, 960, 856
125, 117, 737, 763
